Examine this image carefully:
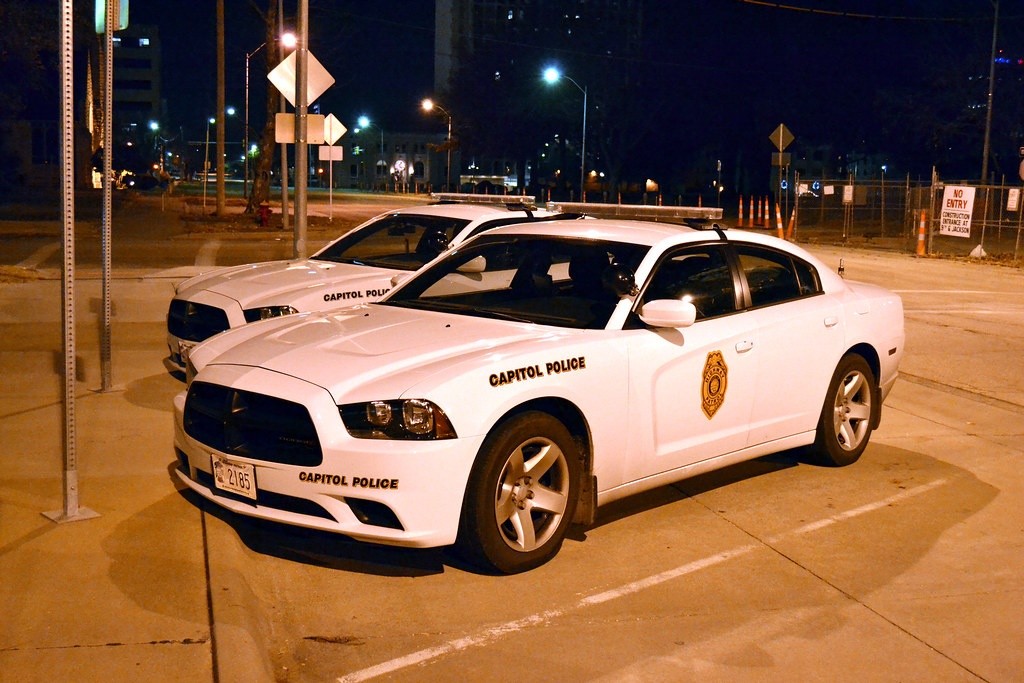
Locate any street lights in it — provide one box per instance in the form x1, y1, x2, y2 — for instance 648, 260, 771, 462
358, 116, 384, 191
543, 68, 587, 203
203, 106, 236, 208
422, 99, 451, 193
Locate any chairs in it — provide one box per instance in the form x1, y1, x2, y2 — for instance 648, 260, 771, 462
553, 245, 613, 320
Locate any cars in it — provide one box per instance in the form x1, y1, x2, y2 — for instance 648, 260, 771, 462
169, 216, 906, 577
164, 201, 602, 376
